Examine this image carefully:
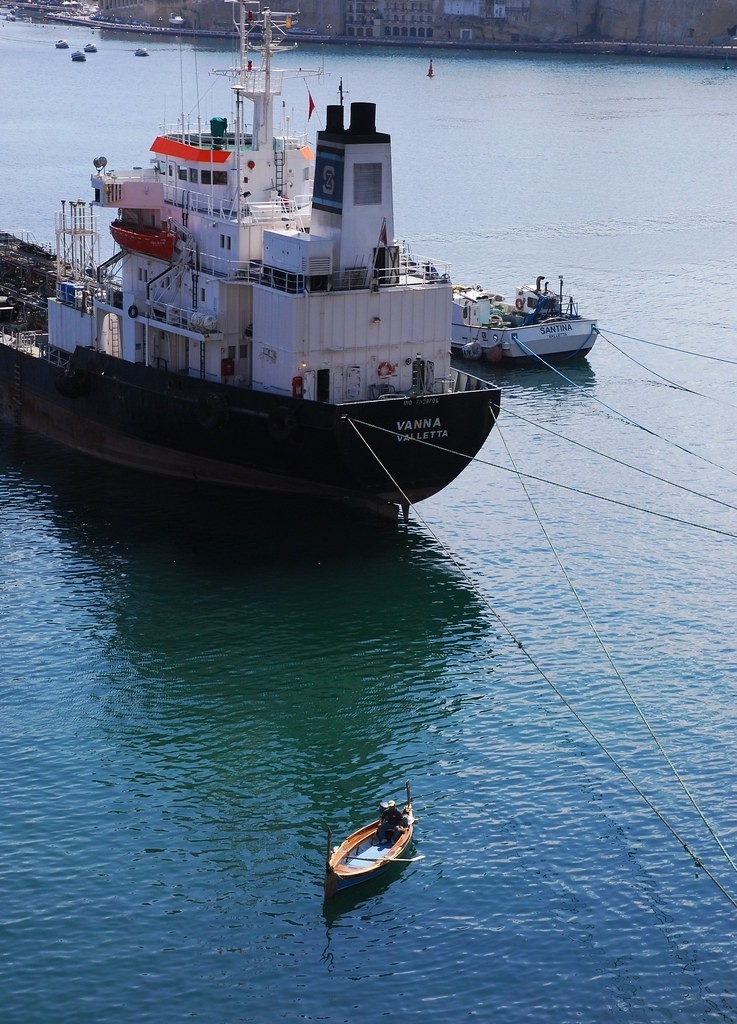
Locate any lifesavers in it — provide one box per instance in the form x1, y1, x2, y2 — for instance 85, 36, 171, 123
378, 361, 393, 379
516, 298, 524, 310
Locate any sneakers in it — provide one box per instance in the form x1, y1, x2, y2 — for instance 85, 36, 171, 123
381, 839, 386, 845
390, 839, 394, 845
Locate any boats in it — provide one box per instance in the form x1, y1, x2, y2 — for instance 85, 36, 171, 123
449, 274, 601, 366
72, 51, 86, 62
84, 44, 97, 52
134, 48, 149, 56
1, 0, 506, 514
56, 40, 69, 48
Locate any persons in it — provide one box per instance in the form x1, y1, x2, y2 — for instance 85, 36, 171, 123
376, 800, 405, 846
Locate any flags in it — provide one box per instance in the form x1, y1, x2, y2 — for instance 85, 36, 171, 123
306, 91, 315, 121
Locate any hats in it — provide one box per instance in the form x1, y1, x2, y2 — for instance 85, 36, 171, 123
388, 799, 394, 807
